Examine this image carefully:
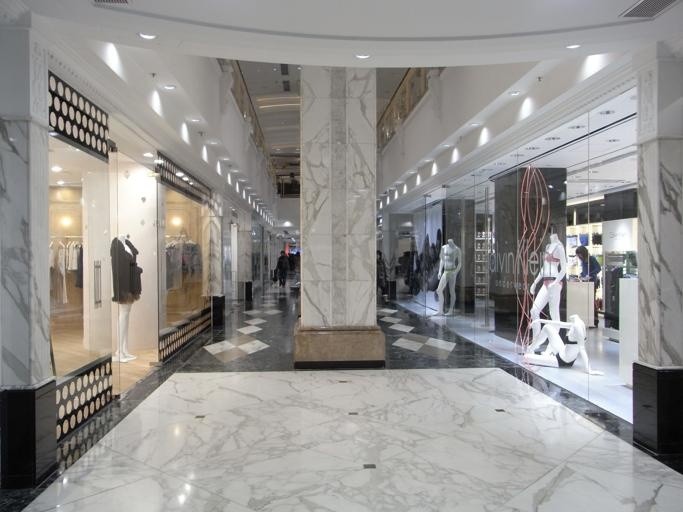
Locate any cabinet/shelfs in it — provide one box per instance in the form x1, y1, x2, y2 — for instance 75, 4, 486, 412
475, 230, 495, 298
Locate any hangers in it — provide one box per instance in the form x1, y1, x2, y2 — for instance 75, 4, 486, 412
49, 239, 82, 249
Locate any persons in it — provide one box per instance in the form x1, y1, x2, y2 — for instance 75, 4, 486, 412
576, 246, 602, 328
433, 239, 462, 317
522, 314, 593, 374
409, 229, 442, 295
529, 233, 567, 342
377, 249, 396, 303
110, 234, 144, 360
275, 250, 291, 287
